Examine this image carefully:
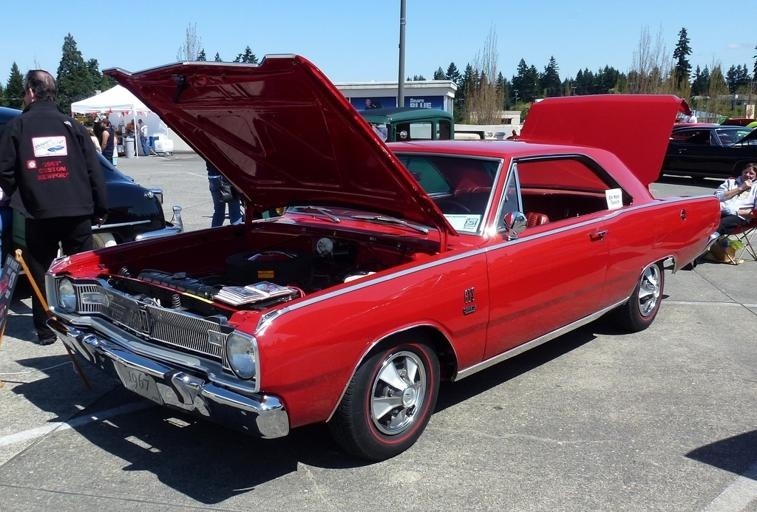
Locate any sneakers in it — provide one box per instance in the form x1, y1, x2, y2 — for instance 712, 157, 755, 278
38, 331, 56, 345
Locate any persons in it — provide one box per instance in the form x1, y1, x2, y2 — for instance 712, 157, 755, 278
204, 161, 242, 227
0, 69, 110, 345
81, 113, 151, 167
683, 110, 696, 123
693, 162, 756, 265
365, 97, 380, 127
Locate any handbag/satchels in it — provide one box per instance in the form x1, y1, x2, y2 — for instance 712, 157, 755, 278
218, 180, 235, 203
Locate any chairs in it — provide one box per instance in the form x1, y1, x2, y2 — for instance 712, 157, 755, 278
525, 211, 549, 231
697, 223, 757, 264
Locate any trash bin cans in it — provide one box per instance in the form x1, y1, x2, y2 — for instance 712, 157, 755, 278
124, 138, 134, 158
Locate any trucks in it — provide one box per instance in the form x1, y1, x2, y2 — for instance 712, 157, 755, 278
261, 101, 455, 221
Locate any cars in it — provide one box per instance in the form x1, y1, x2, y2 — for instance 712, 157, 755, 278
42, 55, 724, 467
657, 116, 757, 180
0, 105, 185, 300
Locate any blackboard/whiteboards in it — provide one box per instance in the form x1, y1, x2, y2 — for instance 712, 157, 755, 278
0, 254, 21, 330
